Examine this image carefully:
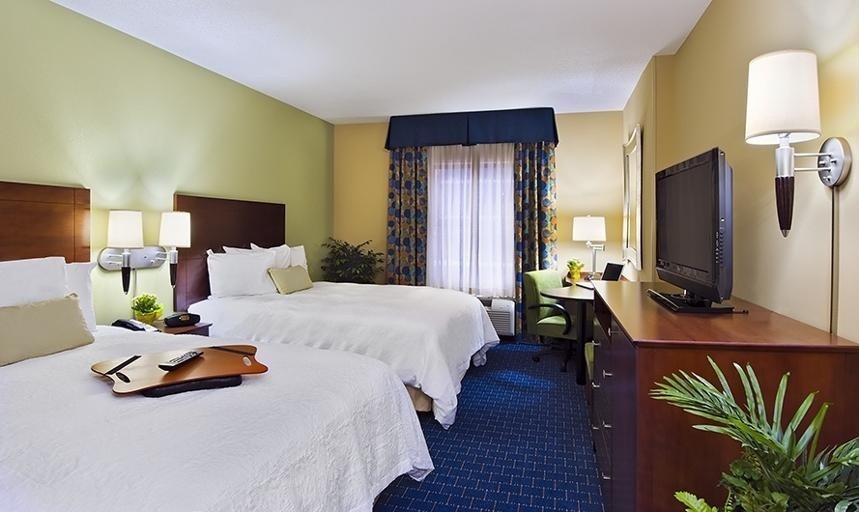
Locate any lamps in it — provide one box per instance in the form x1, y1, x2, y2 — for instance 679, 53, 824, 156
98, 211, 192, 295
573, 215, 606, 280
745, 48, 852, 237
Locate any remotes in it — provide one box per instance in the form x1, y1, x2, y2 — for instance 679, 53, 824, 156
158, 350, 203, 372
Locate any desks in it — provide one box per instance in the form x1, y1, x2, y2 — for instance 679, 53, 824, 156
540, 277, 595, 386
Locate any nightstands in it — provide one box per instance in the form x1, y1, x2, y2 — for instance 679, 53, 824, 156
152, 320, 213, 336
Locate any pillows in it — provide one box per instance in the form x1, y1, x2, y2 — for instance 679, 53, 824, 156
0, 257, 96, 367
207, 245, 313, 296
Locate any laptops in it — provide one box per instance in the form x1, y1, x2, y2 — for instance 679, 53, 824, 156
575, 263, 625, 290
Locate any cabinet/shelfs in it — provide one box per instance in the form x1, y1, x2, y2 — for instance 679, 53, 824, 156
590, 280, 859, 512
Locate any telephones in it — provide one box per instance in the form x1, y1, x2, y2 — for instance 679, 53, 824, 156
111, 319, 161, 332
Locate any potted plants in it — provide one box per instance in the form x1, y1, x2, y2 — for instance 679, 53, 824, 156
131, 293, 163, 325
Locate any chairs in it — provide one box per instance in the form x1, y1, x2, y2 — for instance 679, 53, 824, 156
523, 268, 594, 372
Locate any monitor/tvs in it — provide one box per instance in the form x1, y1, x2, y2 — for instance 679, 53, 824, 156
646, 147, 734, 314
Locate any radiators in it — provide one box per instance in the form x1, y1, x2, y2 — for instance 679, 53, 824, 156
478, 298, 515, 337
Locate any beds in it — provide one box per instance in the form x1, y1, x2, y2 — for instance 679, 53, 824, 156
174, 193, 499, 429
0, 181, 434, 512
